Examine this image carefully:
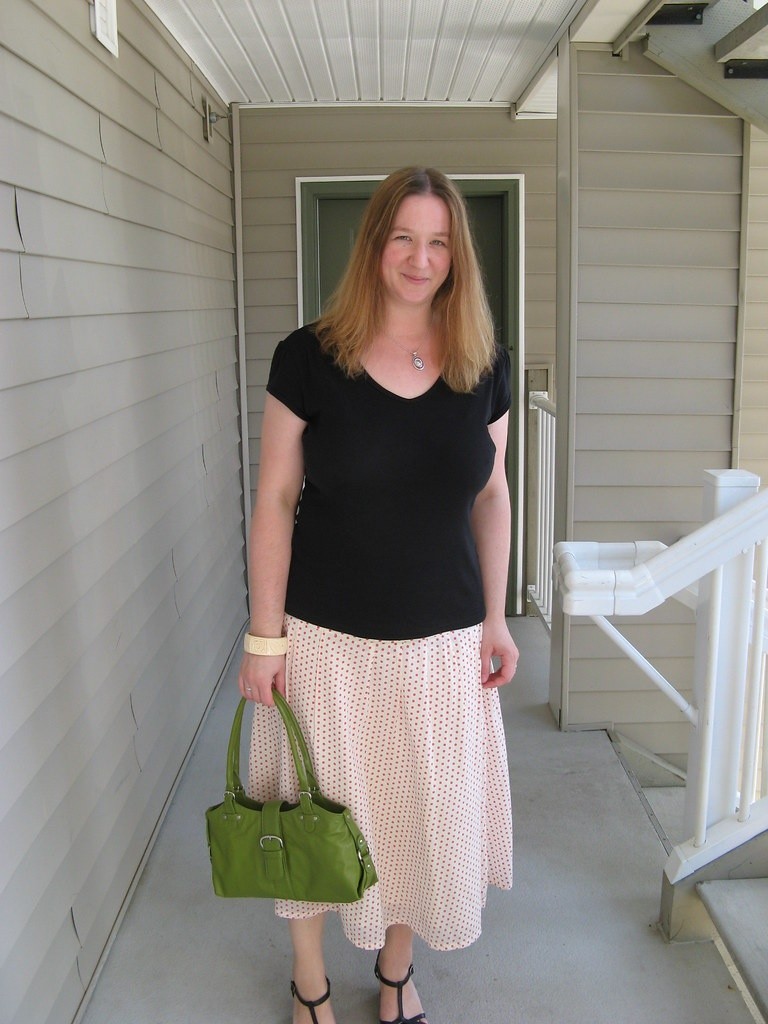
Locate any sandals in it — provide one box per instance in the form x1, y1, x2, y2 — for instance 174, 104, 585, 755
291, 975, 331, 1024
374, 949, 425, 1024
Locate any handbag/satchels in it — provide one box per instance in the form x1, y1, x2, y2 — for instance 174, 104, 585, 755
206, 689, 379, 903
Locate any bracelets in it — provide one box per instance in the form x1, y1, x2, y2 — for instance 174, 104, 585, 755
244, 632, 287, 656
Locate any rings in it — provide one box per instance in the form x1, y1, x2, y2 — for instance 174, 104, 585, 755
246, 687, 250, 691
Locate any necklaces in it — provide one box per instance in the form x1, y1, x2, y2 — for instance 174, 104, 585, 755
382, 323, 431, 370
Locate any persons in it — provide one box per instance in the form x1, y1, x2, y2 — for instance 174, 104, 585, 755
234, 165, 519, 1024
